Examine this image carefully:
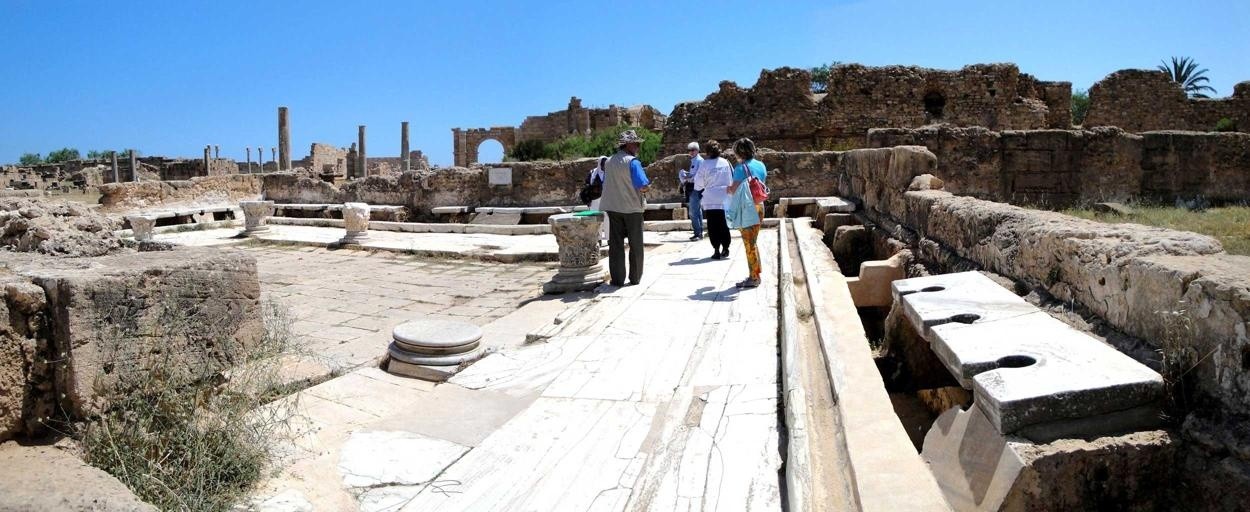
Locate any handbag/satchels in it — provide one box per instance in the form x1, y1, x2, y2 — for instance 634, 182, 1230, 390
750, 177, 771, 203
580, 183, 602, 204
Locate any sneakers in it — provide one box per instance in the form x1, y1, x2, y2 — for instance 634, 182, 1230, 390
711, 249, 729, 259
690, 233, 704, 240
610, 280, 639, 286
736, 277, 760, 287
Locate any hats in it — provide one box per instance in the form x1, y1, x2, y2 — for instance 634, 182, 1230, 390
614, 130, 646, 148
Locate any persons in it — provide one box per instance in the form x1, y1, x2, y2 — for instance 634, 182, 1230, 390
683, 141, 705, 241
599, 131, 651, 286
693, 138, 735, 258
587, 154, 611, 247
725, 137, 767, 289
